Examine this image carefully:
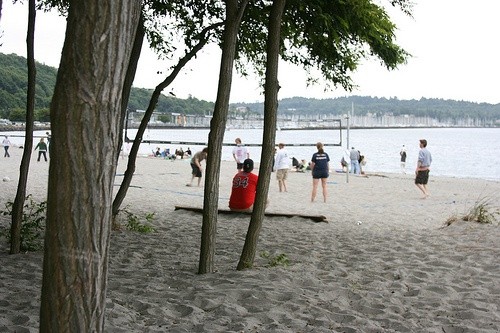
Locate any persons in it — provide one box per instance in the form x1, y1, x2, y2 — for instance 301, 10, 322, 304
415, 140, 432, 199
276, 144, 289, 192
233, 138, 249, 172
191, 148, 208, 187
228, 159, 258, 213
35, 132, 52, 162
272, 145, 406, 174
156, 147, 192, 160
1, 136, 11, 157
311, 143, 329, 203
